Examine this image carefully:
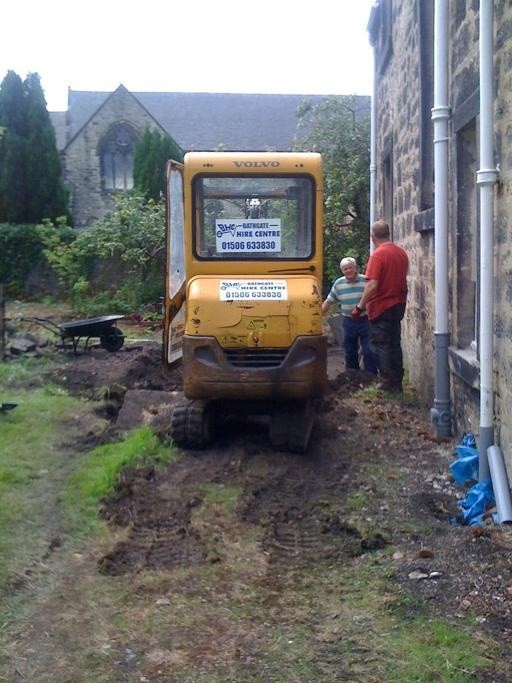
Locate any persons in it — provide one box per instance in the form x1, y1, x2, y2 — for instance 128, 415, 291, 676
352, 219, 409, 396
322, 256, 378, 376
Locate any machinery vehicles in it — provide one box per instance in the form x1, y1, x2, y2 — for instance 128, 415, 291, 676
166, 148, 328, 451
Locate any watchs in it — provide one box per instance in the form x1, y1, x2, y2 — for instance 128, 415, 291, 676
355, 304, 366, 314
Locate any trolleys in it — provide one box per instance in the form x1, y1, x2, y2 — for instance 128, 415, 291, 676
19, 314, 126, 359
